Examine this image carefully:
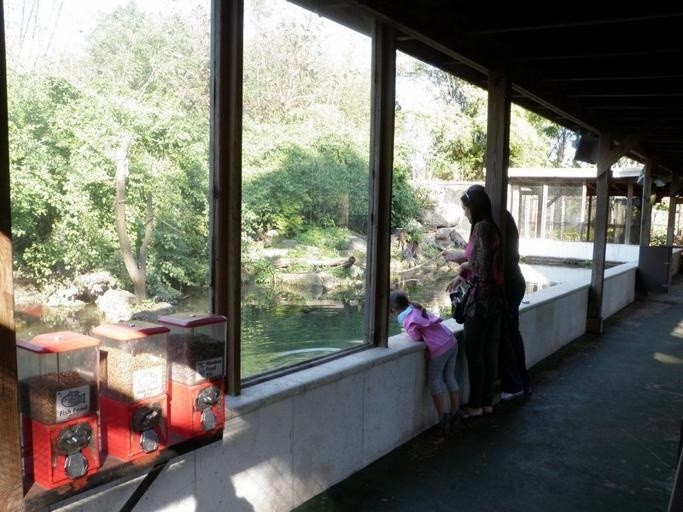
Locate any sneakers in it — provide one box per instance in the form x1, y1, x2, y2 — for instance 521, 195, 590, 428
440, 388, 526, 442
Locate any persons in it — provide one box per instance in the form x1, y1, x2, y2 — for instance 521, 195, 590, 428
389, 290, 466, 434
441, 206, 528, 402
444, 185, 505, 419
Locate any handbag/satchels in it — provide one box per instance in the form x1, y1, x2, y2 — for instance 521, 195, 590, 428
449, 279, 476, 325
